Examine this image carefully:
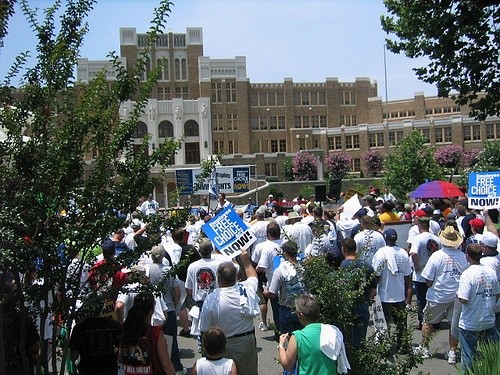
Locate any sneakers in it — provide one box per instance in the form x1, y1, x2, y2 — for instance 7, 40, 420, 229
412, 345, 429, 359
445, 350, 457, 363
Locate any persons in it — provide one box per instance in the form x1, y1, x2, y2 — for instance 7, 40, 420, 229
199, 249, 261, 375
454, 245, 500, 375
278, 294, 351, 375
0, 197, 193, 375
140, 193, 307, 337
404, 197, 500, 365
192, 328, 237, 375
283, 186, 414, 355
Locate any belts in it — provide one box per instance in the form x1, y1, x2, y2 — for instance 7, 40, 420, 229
228, 331, 253, 338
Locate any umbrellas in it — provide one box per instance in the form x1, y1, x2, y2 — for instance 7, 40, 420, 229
410, 181, 465, 199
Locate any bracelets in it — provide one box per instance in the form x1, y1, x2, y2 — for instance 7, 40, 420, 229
277, 345, 285, 351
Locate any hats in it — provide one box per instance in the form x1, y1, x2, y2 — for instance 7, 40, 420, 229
358, 210, 366, 217
383, 228, 397, 241
151, 245, 166, 259
285, 212, 303, 225
447, 214, 455, 220
411, 210, 426, 217
475, 231, 498, 248
438, 226, 463, 247
372, 188, 383, 195
121, 266, 145, 275
416, 216, 429, 224
199, 237, 212, 253
293, 205, 301, 212
469, 218, 484, 229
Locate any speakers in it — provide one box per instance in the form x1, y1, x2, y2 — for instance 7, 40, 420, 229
315, 183, 326, 201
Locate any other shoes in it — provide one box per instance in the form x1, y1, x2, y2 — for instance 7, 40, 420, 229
259, 322, 268, 331
177, 329, 191, 337
176, 368, 188, 375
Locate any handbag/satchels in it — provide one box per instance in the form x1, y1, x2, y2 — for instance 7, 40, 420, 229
283, 365, 298, 375
